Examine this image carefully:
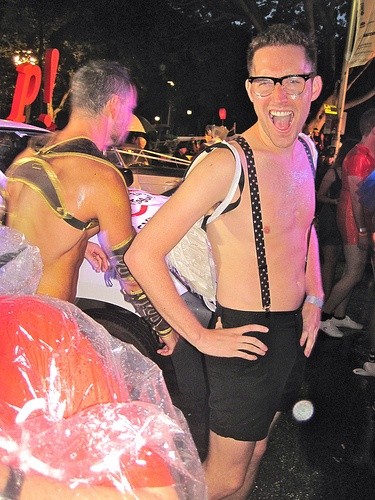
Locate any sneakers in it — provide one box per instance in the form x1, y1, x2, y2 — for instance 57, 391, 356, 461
331, 315, 363, 329
319, 319, 344, 337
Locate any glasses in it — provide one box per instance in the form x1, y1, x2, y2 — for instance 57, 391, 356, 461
248, 72, 316, 97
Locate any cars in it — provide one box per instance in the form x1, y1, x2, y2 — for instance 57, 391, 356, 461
0, 118, 219, 400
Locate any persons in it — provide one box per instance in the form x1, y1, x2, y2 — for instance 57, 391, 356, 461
121, 24, 326, 499
0, 55, 375, 500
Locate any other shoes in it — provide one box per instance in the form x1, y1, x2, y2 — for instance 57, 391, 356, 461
363, 362, 375, 376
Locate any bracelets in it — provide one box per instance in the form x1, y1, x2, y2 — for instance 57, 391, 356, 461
305, 295, 323, 309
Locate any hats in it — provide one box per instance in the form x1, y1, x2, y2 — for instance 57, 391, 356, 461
177, 142, 188, 149
